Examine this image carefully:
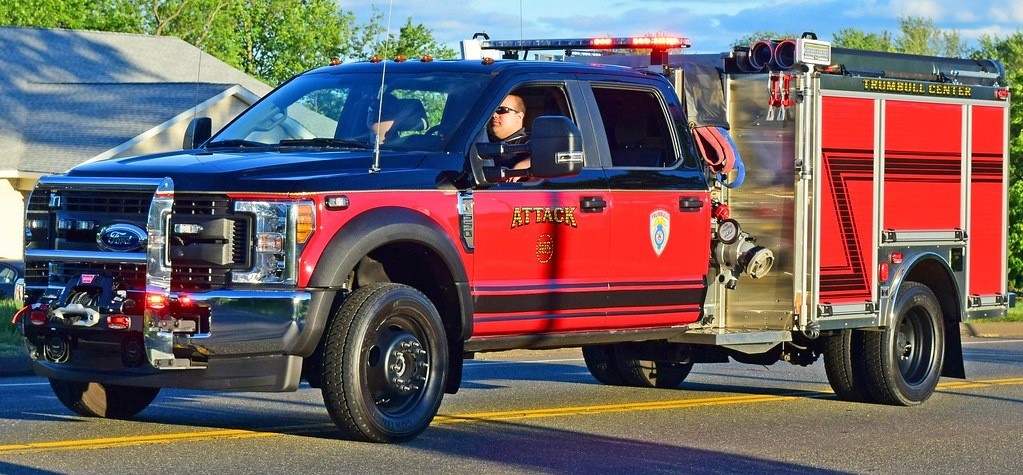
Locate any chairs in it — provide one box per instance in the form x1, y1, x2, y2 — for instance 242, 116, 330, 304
389, 99, 429, 143
612, 117, 666, 167
524, 106, 545, 135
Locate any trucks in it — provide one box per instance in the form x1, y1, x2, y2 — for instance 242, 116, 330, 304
13, 34, 1016, 443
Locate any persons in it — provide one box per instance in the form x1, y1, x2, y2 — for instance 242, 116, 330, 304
489, 95, 531, 183
344, 94, 399, 151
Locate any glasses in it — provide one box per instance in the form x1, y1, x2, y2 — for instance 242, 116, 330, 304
495, 106, 519, 114
368, 106, 390, 113
